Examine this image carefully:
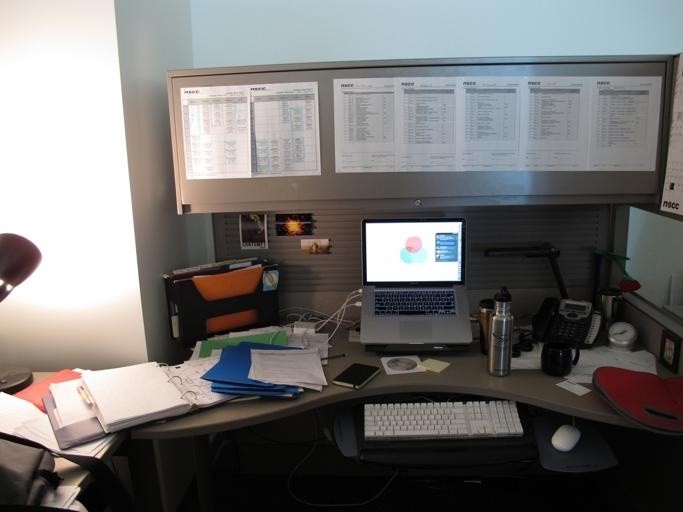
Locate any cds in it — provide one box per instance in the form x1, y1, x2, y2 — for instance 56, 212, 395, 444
386, 357, 417, 372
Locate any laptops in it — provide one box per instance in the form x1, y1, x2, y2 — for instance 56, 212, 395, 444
360, 218, 473, 344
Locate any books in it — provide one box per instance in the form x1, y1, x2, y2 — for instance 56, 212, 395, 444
41, 325, 328, 450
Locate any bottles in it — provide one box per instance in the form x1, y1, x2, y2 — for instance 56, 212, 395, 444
487, 286, 514, 377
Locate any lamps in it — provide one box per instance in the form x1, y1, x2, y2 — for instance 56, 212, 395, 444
483, 244, 570, 299
0, 233, 44, 394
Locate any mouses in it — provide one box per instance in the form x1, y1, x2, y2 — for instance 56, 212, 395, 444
551, 425, 581, 453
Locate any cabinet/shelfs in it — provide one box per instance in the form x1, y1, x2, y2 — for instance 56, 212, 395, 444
166, 53, 674, 215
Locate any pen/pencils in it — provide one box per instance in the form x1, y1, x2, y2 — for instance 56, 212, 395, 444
77, 385, 92, 406
320, 353, 347, 359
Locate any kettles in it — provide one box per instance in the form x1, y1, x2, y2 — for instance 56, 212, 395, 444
598, 288, 625, 339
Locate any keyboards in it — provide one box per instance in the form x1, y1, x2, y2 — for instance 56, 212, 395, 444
364, 400, 524, 441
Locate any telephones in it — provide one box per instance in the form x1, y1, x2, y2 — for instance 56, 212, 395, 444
532, 296, 604, 348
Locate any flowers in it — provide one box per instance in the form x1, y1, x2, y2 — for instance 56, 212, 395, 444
596, 245, 642, 292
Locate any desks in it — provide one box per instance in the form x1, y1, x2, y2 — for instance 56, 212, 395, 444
132, 312, 683, 511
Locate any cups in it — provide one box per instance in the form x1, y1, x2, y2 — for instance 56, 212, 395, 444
541, 341, 580, 377
479, 299, 494, 355
